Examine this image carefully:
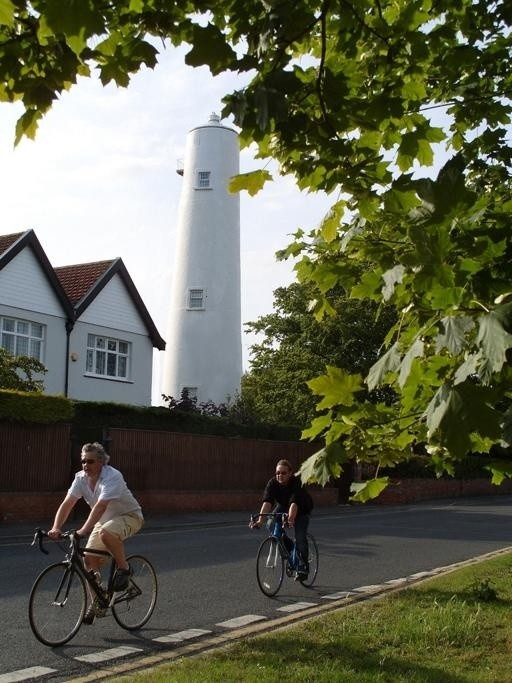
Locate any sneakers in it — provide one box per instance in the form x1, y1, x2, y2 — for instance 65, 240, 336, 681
96, 588, 108, 618
280, 538, 294, 560
113, 563, 134, 591
294, 574, 309, 581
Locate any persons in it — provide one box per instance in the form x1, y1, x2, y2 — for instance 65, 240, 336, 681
253, 460, 313, 581
48, 443, 144, 622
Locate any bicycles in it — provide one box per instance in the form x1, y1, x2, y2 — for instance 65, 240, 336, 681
25, 527, 159, 649
248, 511, 319, 598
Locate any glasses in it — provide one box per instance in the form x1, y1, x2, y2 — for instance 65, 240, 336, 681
275, 470, 288, 476
80, 457, 97, 464
